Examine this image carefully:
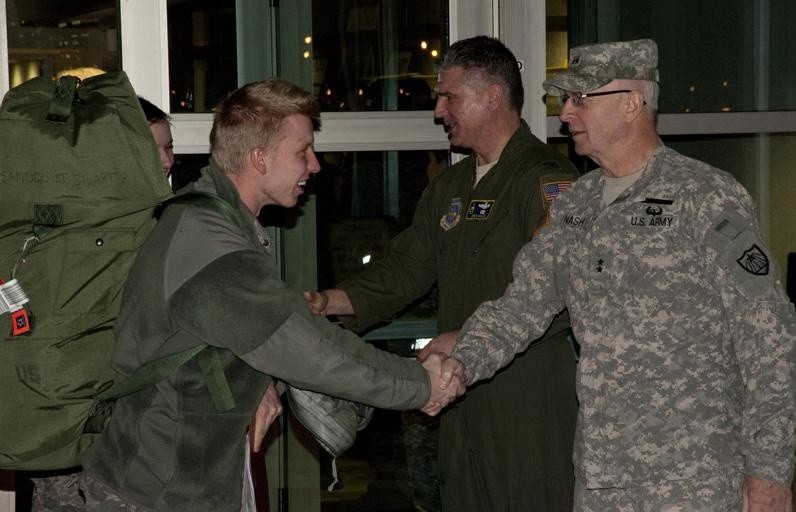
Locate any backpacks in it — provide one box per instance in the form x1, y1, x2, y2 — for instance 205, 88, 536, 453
1, 72, 245, 471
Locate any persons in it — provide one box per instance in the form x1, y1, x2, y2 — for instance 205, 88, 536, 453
76, 74, 471, 512
411, 34, 796, 512
297, 32, 580, 511
28, 92, 178, 512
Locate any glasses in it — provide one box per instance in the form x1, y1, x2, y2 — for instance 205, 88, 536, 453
563, 91, 636, 107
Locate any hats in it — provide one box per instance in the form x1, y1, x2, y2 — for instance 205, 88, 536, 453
542, 40, 659, 93
